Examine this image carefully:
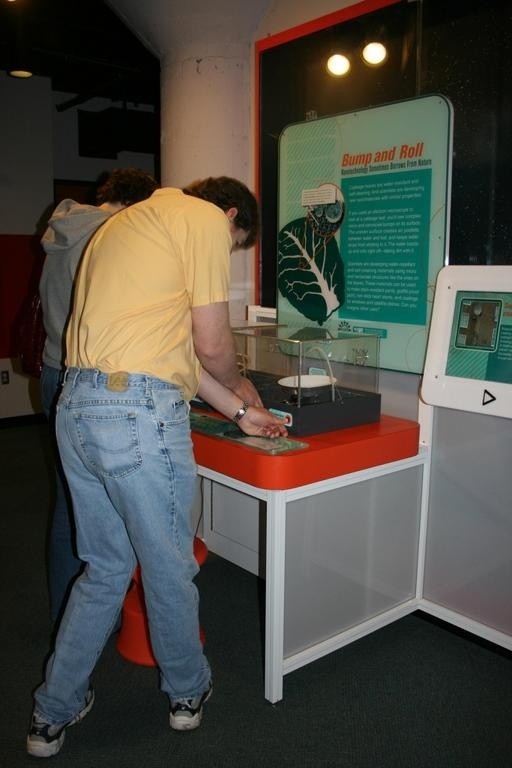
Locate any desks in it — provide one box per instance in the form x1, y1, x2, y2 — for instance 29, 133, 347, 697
193, 414, 431, 708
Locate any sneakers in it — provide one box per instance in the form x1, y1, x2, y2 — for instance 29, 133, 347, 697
170, 678, 214, 732
26, 683, 95, 758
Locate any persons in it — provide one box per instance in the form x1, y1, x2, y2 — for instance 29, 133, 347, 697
25, 172, 290, 758
36, 166, 161, 627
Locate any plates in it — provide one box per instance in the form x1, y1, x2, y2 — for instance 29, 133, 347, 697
278, 374, 337, 389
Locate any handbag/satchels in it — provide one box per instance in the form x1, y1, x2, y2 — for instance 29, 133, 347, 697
14, 291, 47, 379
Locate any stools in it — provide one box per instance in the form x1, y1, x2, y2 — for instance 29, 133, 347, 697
116, 535, 208, 666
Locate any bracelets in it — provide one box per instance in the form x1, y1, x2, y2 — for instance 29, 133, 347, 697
232, 402, 248, 425
